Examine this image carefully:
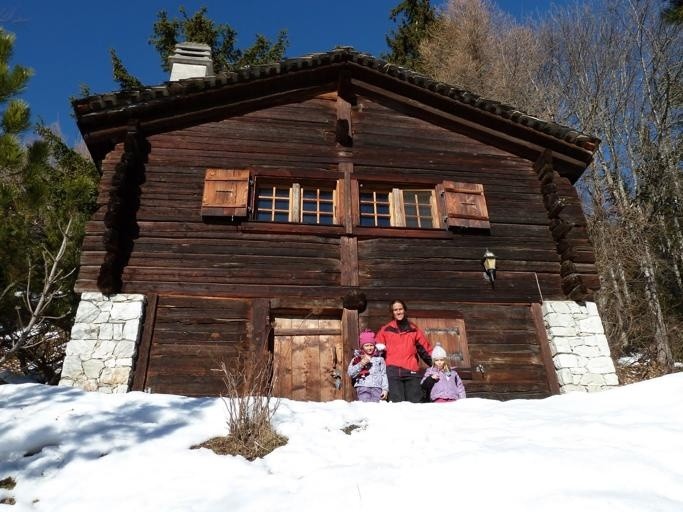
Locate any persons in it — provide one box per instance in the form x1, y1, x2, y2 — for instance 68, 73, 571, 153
346, 328, 389, 402
354, 299, 433, 403
418, 342, 467, 401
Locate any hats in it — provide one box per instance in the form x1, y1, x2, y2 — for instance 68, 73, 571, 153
359, 328, 376, 348
431, 342, 448, 358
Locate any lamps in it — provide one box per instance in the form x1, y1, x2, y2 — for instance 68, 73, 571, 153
482, 248, 498, 282
